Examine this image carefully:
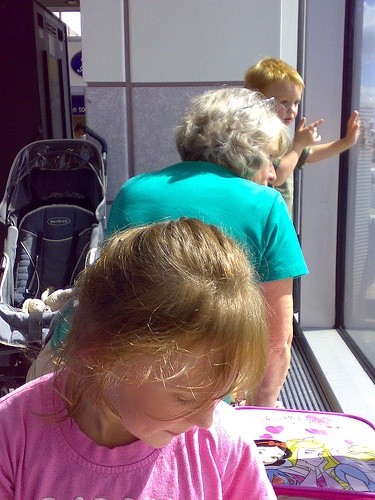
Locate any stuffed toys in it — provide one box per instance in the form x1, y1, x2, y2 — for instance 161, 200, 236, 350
20, 286, 76, 314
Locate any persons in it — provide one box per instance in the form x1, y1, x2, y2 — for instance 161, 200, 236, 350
101, 85, 311, 409
73, 121, 86, 140
0, 213, 283, 500
244, 56, 360, 225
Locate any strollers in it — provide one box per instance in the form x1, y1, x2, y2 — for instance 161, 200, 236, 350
1, 125, 107, 383
